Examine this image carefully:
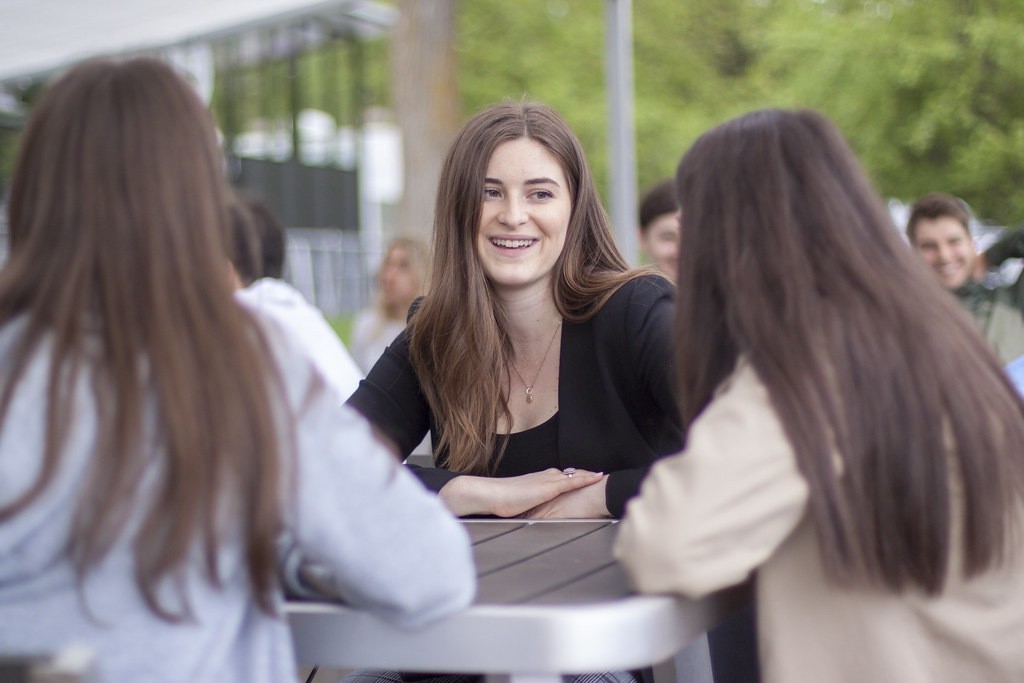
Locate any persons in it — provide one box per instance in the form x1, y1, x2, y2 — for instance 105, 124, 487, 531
905, 194, 1023, 370
343, 94, 687, 520
639, 179, 683, 284
614, 107, 1023, 683
228, 192, 369, 405
345, 238, 429, 378
0, 56, 477, 683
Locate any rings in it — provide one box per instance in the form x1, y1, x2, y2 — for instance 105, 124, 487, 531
563, 468, 577, 478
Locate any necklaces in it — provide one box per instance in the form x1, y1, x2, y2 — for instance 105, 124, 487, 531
508, 316, 563, 404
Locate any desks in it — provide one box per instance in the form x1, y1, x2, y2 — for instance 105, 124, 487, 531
284, 515, 736, 682
